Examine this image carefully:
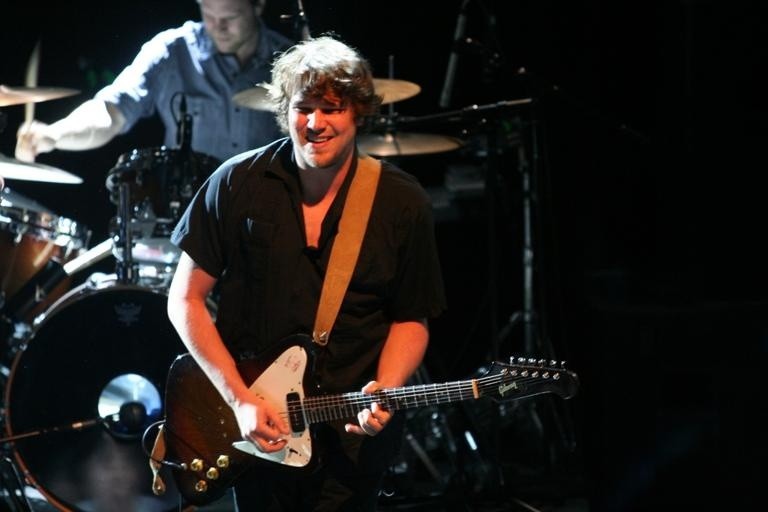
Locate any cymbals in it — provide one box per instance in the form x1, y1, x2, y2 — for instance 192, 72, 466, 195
231, 78, 421, 112
353, 131, 464, 156
0, 153, 84, 185
0, 83, 83, 106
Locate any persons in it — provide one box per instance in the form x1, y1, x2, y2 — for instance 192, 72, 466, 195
165, 37, 441, 512
16, 0, 291, 234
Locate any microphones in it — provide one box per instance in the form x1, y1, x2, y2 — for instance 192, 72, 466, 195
118, 400, 149, 433
438, 11, 472, 111
172, 95, 188, 144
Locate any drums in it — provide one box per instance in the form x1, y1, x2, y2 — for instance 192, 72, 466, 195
4, 270, 218, 512
107, 145, 224, 264
0, 187, 93, 340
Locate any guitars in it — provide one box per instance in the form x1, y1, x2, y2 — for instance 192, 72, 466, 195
164, 333, 580, 506
